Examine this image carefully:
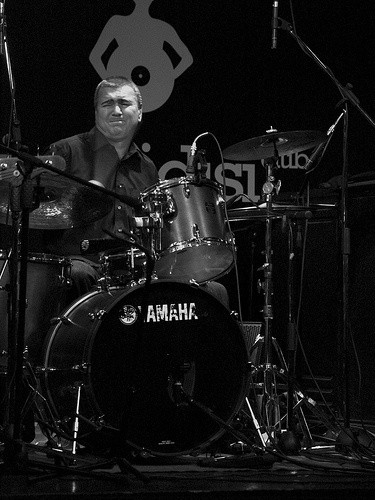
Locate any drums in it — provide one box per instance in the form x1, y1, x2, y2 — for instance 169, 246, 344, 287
223, 218, 297, 322
134, 175, 237, 287
26, 275, 250, 459
18, 253, 109, 385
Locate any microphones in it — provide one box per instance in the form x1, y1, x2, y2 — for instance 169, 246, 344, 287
186, 142, 197, 173
0, 0, 6, 54
225, 195, 241, 207
172, 380, 197, 403
304, 141, 326, 170
271, 0, 279, 49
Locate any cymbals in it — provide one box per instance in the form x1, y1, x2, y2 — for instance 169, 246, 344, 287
222, 130, 330, 161
225, 205, 319, 218
0, 162, 115, 230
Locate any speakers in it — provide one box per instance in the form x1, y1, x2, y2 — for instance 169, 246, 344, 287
239, 321, 265, 367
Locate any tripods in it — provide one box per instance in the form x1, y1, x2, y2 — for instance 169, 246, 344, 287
249, 17, 375, 460
0, 142, 142, 485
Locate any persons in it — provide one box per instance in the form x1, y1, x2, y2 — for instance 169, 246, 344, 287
25, 77, 229, 370
